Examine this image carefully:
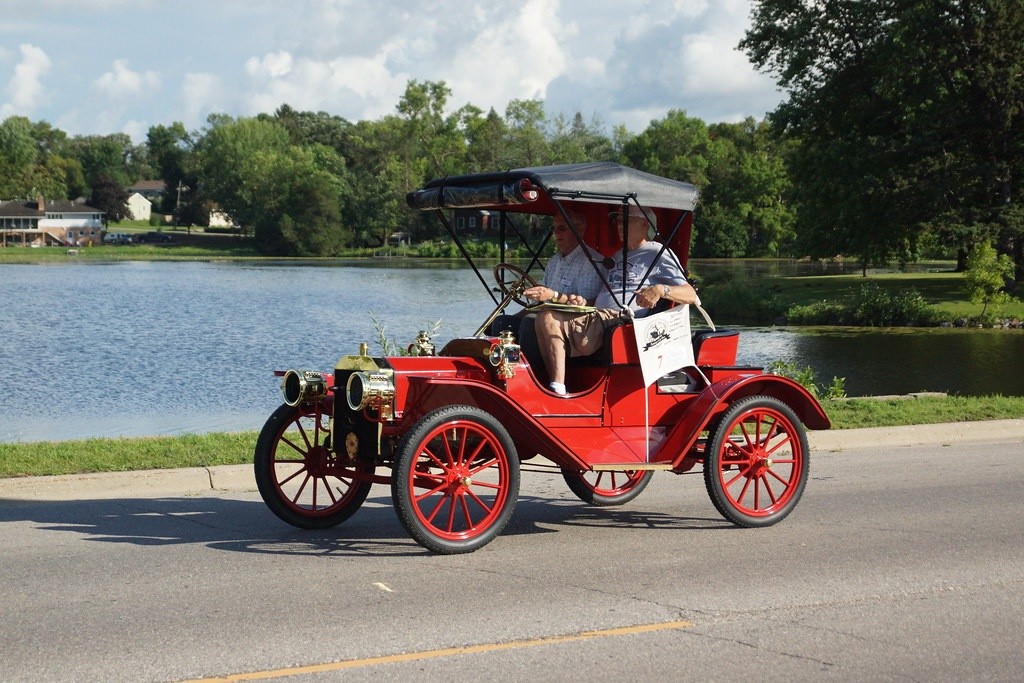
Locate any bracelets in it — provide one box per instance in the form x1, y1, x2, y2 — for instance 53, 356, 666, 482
661, 284, 670, 299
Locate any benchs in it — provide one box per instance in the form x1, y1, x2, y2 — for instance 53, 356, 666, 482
569, 298, 668, 368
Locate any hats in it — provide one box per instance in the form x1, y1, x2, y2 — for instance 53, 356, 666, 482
607, 205, 648, 219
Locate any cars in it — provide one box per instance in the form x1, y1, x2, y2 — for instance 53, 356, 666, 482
253, 159, 831, 554
104, 231, 172, 245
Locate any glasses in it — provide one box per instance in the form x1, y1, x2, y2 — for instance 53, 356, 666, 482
613, 218, 640, 224
549, 225, 570, 232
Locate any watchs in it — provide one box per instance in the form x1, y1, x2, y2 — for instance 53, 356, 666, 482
550, 292, 562, 303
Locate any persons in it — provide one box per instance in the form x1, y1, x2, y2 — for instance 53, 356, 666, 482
492, 205, 609, 391
535, 205, 697, 395
